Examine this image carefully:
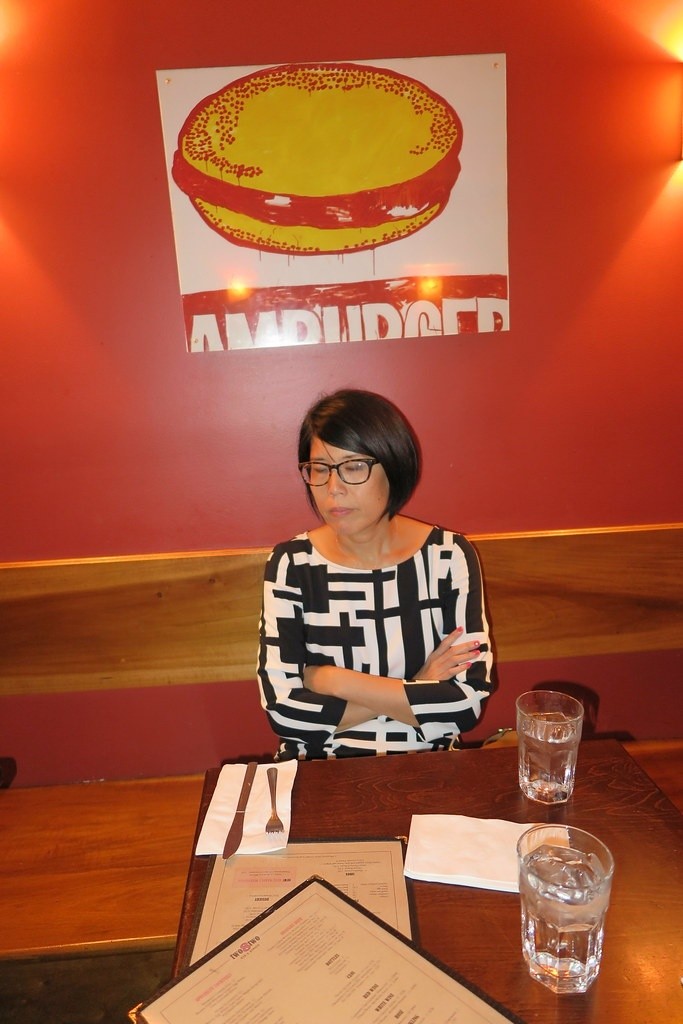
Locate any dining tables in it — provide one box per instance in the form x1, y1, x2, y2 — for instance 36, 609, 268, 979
173, 740, 683, 1024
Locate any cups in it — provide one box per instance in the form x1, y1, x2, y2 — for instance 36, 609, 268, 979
514, 690, 584, 804
516, 823, 614, 994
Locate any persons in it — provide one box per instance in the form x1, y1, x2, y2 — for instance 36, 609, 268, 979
258, 390, 501, 762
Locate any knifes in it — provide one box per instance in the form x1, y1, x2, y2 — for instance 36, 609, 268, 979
222, 762, 258, 860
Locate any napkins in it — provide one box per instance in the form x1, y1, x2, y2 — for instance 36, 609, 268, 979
194, 759, 298, 856
403, 814, 569, 894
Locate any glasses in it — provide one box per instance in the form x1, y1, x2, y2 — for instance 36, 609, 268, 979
298, 458, 380, 487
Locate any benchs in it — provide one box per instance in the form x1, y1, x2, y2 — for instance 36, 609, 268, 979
0, 524, 683, 958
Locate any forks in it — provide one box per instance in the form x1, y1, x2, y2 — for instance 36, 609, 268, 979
265, 767, 285, 835
